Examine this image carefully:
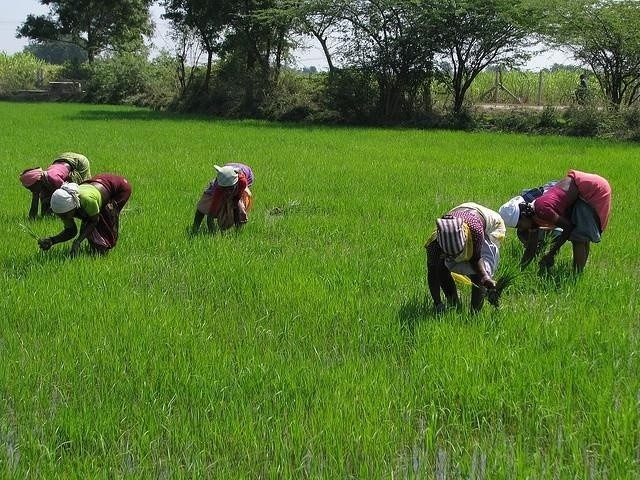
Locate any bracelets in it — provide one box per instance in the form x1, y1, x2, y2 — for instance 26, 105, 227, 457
480, 274, 491, 285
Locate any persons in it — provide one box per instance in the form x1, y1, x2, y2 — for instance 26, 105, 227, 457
574, 74, 587, 106
192, 161, 254, 235
501, 167, 612, 277
423, 201, 508, 314
36, 173, 133, 257
509, 181, 564, 256
19, 151, 92, 219
